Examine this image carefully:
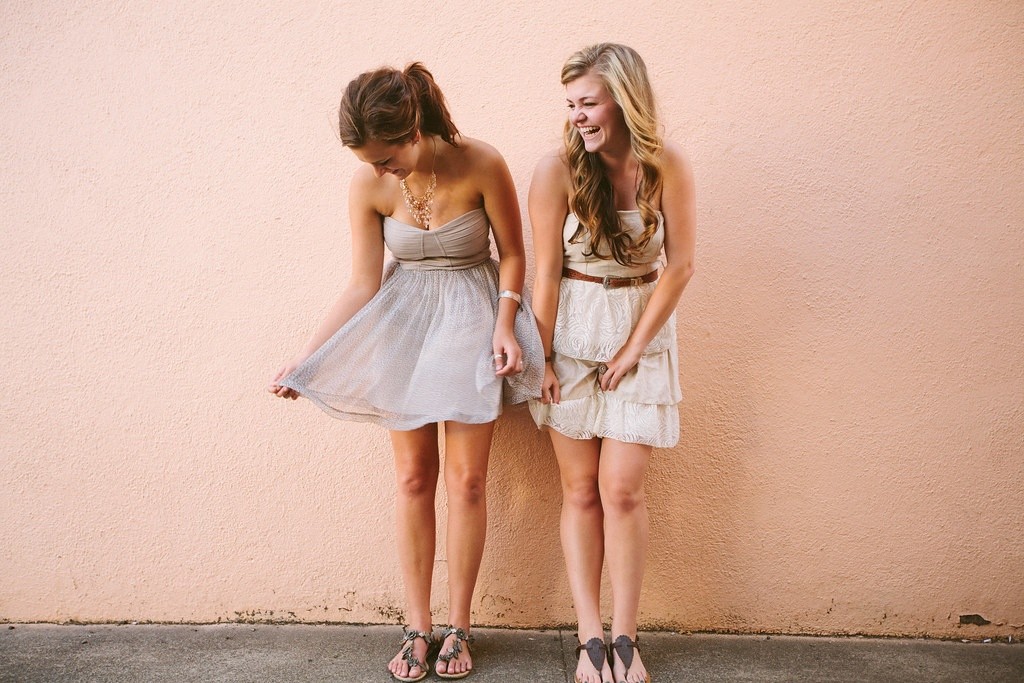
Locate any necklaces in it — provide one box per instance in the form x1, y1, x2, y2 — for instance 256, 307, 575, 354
398, 134, 437, 227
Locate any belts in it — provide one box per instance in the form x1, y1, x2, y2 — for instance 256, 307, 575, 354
562, 267, 658, 291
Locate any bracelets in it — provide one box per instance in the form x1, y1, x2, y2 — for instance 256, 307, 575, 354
495, 290, 522, 306
545, 357, 553, 363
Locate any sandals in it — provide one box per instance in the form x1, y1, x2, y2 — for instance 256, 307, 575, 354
610, 635, 651, 683
573, 632, 615, 683
390, 624, 435, 682
435, 624, 472, 678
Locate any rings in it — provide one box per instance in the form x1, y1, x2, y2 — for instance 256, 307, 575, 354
598, 362, 607, 373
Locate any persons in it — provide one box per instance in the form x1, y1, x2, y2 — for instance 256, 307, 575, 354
526, 40, 697, 683
267, 62, 545, 681
